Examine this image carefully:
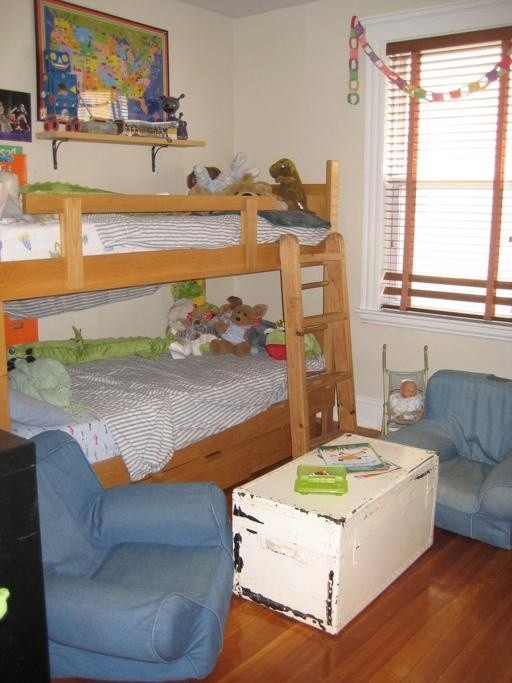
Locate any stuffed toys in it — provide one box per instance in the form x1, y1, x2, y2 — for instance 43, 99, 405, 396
268, 157, 309, 214
7, 325, 172, 366
207, 150, 261, 193
185, 172, 276, 217
161, 280, 322, 367
186, 164, 221, 188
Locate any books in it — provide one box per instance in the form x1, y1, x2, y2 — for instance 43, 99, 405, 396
353, 457, 401, 480
318, 442, 389, 474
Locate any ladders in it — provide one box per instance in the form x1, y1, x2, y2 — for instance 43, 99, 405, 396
275, 231, 359, 458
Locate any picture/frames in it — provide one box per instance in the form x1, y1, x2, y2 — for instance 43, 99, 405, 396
34, 0, 169, 123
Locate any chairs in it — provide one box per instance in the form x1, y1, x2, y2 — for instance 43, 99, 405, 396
379, 369, 512, 550
27, 430, 233, 682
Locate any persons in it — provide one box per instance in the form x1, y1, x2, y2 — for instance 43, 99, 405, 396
388, 379, 426, 423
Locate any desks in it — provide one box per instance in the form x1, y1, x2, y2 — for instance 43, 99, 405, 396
232, 434, 439, 633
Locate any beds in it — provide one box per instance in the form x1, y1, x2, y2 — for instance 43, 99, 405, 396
0, 161, 358, 492
